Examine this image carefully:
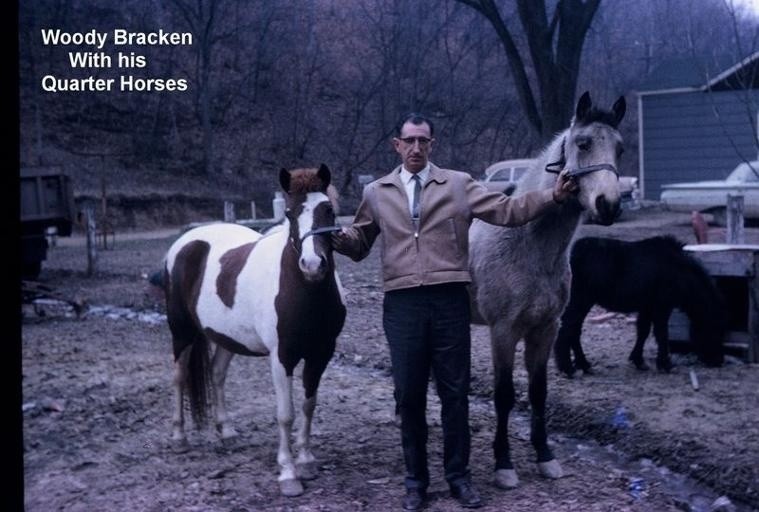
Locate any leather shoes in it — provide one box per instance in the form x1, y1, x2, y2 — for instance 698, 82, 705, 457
448, 483, 483, 509
402, 489, 427, 511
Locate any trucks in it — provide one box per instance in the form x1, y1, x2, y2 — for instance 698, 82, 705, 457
19, 162, 75, 279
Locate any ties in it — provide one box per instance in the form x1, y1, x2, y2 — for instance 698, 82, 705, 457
409, 175, 421, 220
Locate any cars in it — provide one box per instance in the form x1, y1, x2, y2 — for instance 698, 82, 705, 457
478, 158, 639, 204
658, 159, 759, 225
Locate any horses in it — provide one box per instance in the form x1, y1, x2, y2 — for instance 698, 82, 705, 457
554, 232, 731, 373
162, 163, 347, 497
454, 91, 627, 489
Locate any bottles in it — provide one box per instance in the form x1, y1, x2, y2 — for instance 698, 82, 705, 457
691, 207, 709, 243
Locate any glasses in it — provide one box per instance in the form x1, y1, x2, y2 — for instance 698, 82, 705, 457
400, 137, 430, 145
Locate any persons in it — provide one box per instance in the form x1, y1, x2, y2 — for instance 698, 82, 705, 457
330, 112, 579, 510
